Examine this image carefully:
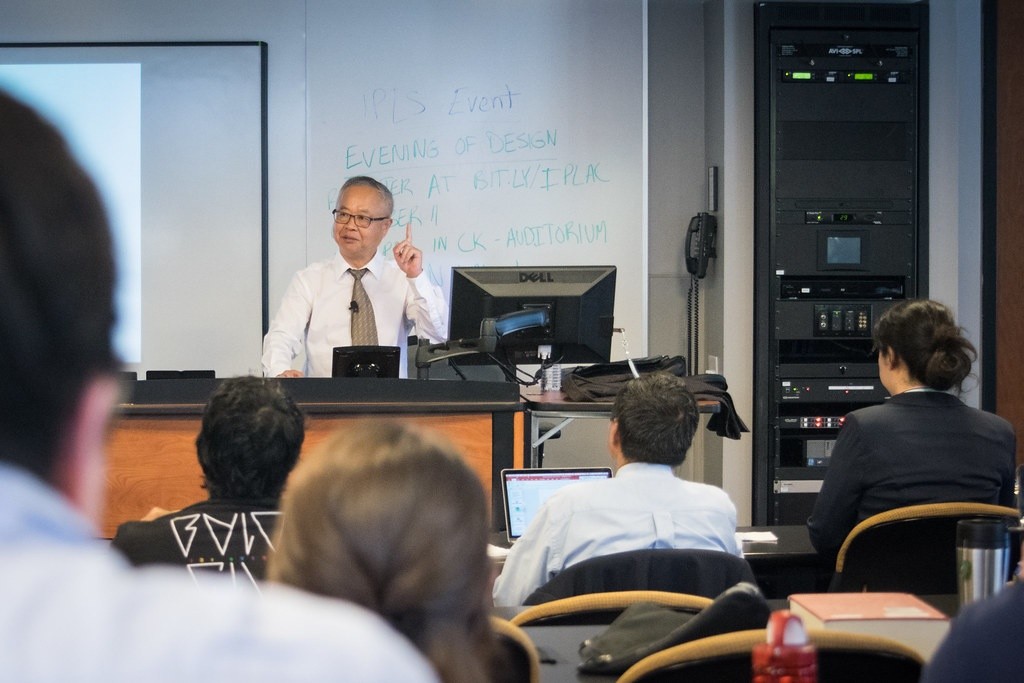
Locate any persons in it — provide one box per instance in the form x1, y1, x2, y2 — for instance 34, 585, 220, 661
109, 377, 306, 582
1, 91, 435, 683
260, 176, 449, 379
808, 299, 1016, 588
261, 421, 495, 683
491, 373, 742, 622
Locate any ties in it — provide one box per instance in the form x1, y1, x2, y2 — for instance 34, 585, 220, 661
345, 266, 379, 347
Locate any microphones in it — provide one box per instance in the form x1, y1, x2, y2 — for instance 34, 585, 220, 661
351, 300, 359, 311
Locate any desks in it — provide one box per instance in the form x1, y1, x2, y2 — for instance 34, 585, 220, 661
99, 377, 532, 537
522, 387, 725, 490
734, 524, 828, 597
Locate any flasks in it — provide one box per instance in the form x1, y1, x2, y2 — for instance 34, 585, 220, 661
956, 519, 1011, 607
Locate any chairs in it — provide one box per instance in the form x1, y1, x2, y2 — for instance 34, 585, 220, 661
145, 369, 215, 380
117, 370, 138, 381
523, 548, 757, 607
830, 500, 1024, 597
611, 625, 927, 683
510, 589, 715, 627
489, 616, 541, 683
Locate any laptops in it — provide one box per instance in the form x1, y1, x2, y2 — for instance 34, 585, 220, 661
501, 467, 613, 547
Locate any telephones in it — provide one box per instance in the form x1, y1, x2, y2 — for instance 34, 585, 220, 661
685, 212, 717, 279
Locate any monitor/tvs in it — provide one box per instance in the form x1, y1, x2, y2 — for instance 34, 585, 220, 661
331, 346, 400, 380
448, 265, 617, 366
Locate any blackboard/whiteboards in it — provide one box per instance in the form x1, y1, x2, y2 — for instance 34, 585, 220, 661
0, 0, 650, 378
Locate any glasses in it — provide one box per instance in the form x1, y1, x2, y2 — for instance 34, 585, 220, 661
330, 208, 388, 227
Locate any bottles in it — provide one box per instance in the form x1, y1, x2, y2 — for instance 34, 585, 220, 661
752, 609, 817, 683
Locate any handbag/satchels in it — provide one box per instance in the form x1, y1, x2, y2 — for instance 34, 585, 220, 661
575, 581, 770, 683
